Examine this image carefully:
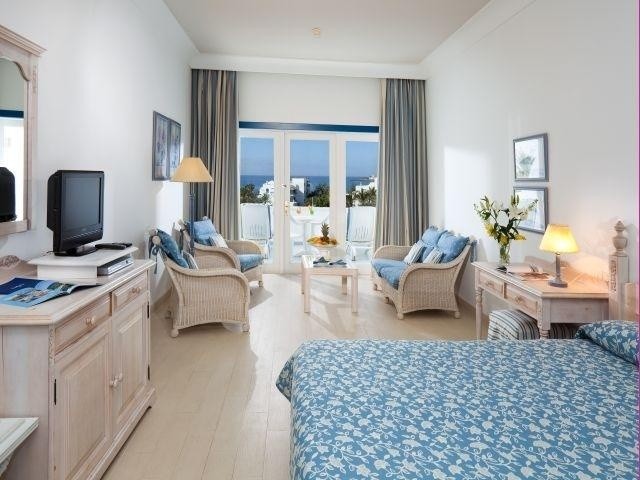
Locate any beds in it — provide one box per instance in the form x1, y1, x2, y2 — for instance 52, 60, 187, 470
291, 219, 640, 480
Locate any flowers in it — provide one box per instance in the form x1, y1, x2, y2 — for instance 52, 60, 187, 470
473, 188, 539, 262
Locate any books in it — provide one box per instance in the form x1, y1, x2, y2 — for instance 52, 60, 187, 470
497, 263, 556, 281
0, 274, 105, 309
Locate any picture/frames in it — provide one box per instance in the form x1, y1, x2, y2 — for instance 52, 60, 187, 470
152, 111, 182, 181
512, 133, 549, 181
511, 185, 548, 234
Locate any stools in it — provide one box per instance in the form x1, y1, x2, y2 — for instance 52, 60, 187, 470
488, 308, 579, 340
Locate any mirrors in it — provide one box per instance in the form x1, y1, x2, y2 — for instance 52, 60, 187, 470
0, 24, 47, 237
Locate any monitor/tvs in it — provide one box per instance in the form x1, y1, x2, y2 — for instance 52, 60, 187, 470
46, 169, 105, 257
0, 167, 17, 223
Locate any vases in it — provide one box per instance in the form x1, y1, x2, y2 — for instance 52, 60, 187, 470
498, 243, 511, 267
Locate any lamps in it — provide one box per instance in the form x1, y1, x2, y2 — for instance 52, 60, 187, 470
538, 224, 579, 288
170, 156, 214, 257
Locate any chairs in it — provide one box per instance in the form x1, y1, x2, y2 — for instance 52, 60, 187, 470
149, 215, 264, 338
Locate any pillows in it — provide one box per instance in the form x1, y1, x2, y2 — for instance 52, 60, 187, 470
575, 320, 639, 364
403, 224, 469, 266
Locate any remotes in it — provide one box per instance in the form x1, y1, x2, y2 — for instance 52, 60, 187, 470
112, 243, 132, 247
95, 243, 126, 250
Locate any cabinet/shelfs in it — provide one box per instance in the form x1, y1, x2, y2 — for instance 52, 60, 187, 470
470, 260, 610, 340
0, 259, 158, 480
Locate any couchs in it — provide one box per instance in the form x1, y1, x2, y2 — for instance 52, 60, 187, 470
370, 232, 475, 320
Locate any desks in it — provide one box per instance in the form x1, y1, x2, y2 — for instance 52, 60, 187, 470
0, 415, 40, 479
